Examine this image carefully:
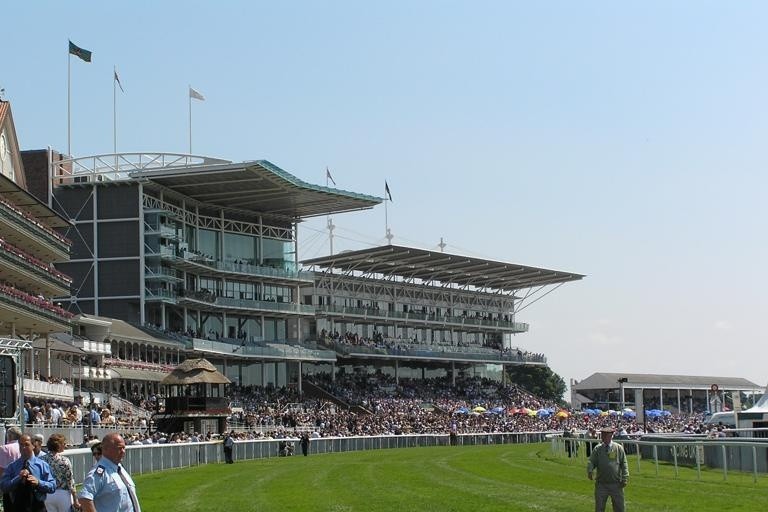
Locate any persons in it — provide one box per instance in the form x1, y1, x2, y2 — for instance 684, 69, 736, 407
587, 427, 630, 512
16, 371, 235, 464
564, 427, 641, 458
0, 433, 57, 512
321, 329, 383, 350
569, 409, 726, 437
302, 372, 452, 437
451, 376, 568, 434
38, 434, 82, 512
147, 323, 195, 339
235, 382, 310, 457
78, 432, 141, 512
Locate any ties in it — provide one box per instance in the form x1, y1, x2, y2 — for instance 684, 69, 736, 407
118, 467, 138, 512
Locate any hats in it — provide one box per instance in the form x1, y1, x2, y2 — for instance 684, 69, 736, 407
601, 427, 614, 432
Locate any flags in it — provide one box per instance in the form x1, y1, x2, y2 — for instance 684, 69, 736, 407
189, 84, 206, 102
385, 180, 393, 203
114, 67, 125, 95
69, 39, 92, 63
326, 166, 336, 187
